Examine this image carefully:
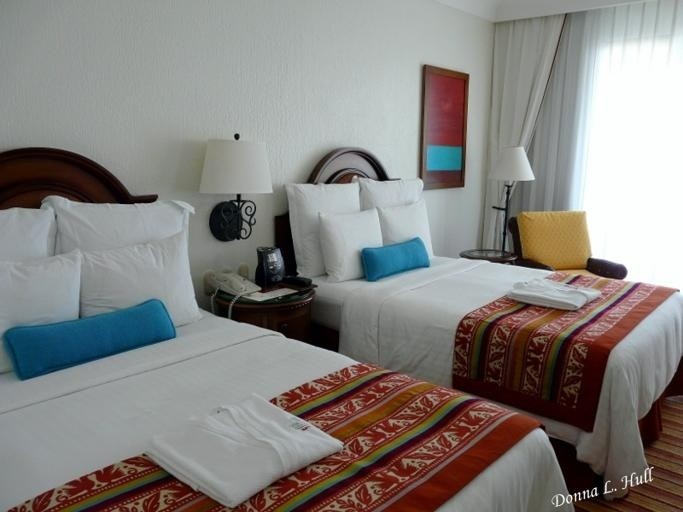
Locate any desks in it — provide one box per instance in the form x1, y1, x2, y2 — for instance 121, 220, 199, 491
456, 246, 517, 264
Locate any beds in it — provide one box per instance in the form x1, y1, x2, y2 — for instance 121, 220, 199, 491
0, 143, 576, 511
270, 145, 680, 501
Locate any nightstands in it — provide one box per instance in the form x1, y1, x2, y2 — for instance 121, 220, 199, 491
208, 275, 316, 342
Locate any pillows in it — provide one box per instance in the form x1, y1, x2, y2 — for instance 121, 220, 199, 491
283, 174, 435, 284
1, 191, 205, 382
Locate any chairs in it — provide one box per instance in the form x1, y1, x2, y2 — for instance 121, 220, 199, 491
506, 208, 628, 281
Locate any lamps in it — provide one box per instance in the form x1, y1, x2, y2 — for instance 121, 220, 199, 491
199, 132, 275, 244
486, 145, 534, 260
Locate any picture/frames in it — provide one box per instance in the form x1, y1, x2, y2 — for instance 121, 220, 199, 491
416, 63, 469, 192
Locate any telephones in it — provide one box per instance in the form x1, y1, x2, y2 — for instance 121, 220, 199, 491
203, 269, 262, 296
